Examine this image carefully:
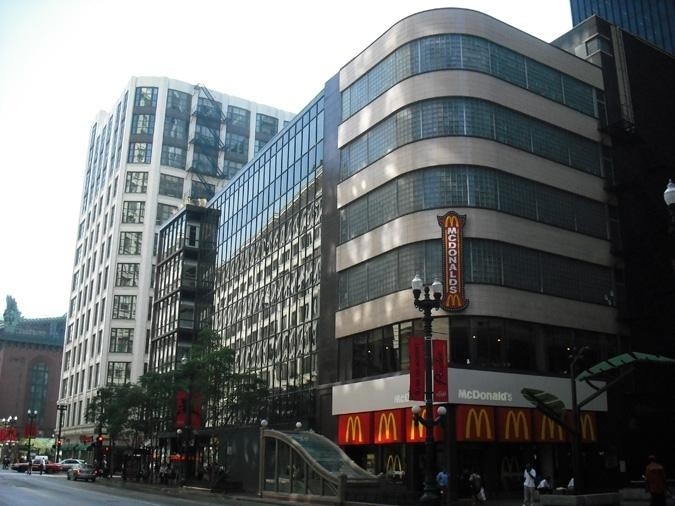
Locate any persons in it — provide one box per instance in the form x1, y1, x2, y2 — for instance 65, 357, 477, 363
535, 475, 554, 496
522, 462, 538, 506
645, 454, 668, 506
435, 464, 450, 506
468, 470, 484, 506
139, 461, 169, 486
1, 454, 43, 476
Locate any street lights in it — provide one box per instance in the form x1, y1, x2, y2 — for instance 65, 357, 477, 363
664, 180, 675, 220
176, 354, 199, 484
1, 402, 67, 463
411, 272, 447, 505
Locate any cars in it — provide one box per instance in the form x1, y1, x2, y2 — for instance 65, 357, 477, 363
10, 459, 87, 474
67, 464, 96, 482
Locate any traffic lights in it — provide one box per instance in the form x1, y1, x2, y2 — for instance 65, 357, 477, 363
96, 436, 103, 447
